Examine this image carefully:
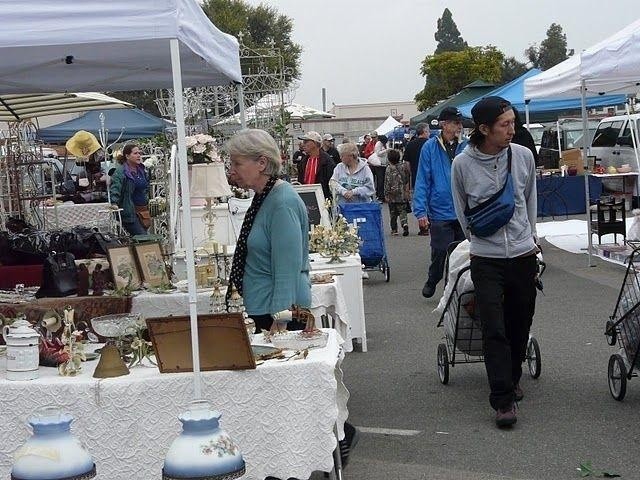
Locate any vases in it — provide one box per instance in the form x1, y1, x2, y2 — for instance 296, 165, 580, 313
56, 354, 83, 377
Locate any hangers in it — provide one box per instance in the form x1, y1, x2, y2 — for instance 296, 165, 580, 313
98, 112, 125, 234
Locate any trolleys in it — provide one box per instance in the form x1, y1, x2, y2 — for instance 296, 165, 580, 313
435, 241, 546, 384
332, 182, 390, 282
604, 247, 640, 400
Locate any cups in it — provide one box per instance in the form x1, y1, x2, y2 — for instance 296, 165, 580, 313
16, 284, 24, 303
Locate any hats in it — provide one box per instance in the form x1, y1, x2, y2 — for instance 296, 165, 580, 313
297, 131, 322, 143
323, 134, 333, 141
65, 129, 100, 158
437, 106, 471, 122
467, 96, 512, 137
368, 130, 378, 138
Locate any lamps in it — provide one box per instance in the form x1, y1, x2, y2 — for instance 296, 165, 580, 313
190, 162, 226, 252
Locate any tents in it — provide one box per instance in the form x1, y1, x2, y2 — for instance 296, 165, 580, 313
35, 108, 176, 195
0, 0, 247, 403
524, 19, 640, 270
212, 93, 336, 126
410, 79, 497, 130
0, 93, 137, 123
457, 68, 640, 170
360, 115, 403, 142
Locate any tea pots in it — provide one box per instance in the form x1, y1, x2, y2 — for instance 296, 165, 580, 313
3, 320, 33, 343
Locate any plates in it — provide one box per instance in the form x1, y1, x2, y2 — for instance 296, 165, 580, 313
617, 167, 632, 173
90, 313, 142, 337
271, 332, 329, 349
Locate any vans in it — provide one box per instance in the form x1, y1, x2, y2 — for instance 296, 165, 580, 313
538, 119, 600, 170
589, 114, 640, 198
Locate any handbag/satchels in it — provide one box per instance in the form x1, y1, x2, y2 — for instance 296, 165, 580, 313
35, 231, 77, 298
137, 211, 152, 228
396, 166, 412, 214
463, 143, 516, 237
88, 232, 122, 254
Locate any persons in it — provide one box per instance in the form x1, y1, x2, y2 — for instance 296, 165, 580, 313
374, 135, 388, 200
364, 131, 376, 158
329, 143, 376, 203
293, 139, 307, 163
110, 144, 150, 236
225, 128, 312, 334
298, 131, 334, 198
403, 123, 431, 188
360, 135, 371, 157
383, 150, 410, 235
413, 106, 470, 297
320, 134, 342, 163
451, 96, 541, 425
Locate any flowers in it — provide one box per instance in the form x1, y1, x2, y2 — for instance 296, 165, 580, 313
185, 133, 222, 164
309, 198, 362, 254
59, 321, 83, 353
122, 313, 159, 368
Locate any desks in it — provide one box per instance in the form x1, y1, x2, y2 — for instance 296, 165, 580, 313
536, 168, 602, 217
177, 203, 237, 246
38, 201, 109, 233
0, 252, 368, 353
0, 328, 351, 479
590, 171, 640, 213
0, 268, 354, 356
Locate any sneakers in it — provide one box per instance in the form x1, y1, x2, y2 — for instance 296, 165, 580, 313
422, 276, 443, 298
361, 264, 369, 279
391, 230, 399, 236
323, 422, 360, 478
402, 223, 409, 236
418, 227, 429, 235
495, 399, 517, 425
513, 383, 524, 401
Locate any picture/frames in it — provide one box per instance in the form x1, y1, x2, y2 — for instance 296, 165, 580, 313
293, 183, 332, 229
107, 246, 143, 290
133, 240, 172, 288
145, 312, 256, 374
